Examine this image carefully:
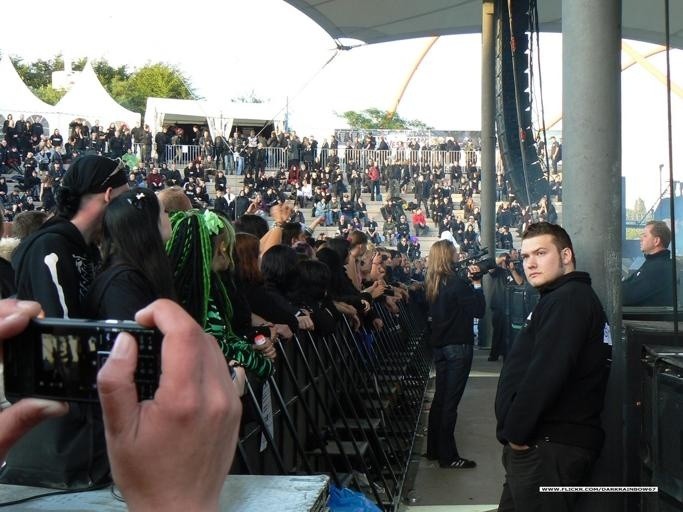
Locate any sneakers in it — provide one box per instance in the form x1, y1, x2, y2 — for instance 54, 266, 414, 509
488, 355, 498, 361
441, 458, 476, 468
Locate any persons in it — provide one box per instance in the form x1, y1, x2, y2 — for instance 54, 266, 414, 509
1, 121, 562, 399
425, 240, 486, 469
622, 220, 673, 307
494, 222, 613, 512
0, 296, 244, 512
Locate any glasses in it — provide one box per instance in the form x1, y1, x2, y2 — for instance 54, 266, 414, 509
102, 158, 125, 186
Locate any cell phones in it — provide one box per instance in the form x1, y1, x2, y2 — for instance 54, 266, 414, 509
3, 318, 163, 406
